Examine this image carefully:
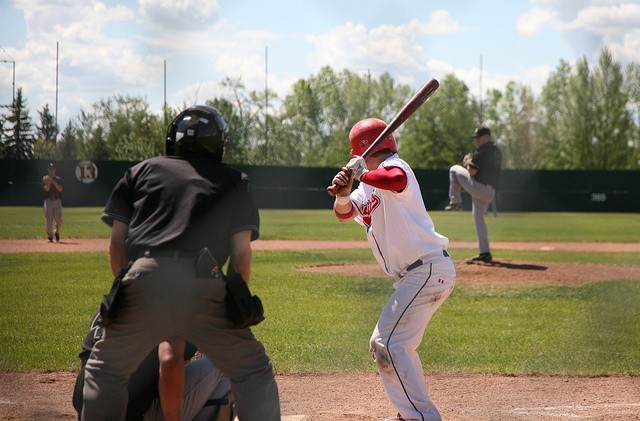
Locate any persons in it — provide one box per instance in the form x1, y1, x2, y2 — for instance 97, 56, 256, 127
72, 311, 238, 421
445, 125, 502, 263
78, 106, 282, 421
334, 118, 456, 421
41, 162, 64, 243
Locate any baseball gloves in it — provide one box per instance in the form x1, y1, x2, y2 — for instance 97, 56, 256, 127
43, 175, 52, 183
463, 153, 473, 167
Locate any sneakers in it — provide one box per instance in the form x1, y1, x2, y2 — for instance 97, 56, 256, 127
444, 203, 463, 210
471, 253, 492, 261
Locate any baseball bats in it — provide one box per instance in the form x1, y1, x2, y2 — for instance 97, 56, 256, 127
327, 78, 438, 196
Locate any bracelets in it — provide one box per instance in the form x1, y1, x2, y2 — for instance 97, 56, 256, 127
335, 205, 354, 219
336, 194, 350, 205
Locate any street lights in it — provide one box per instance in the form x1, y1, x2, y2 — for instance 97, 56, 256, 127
2, 56, 18, 150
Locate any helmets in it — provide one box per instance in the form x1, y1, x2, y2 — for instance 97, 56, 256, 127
165, 105, 229, 160
348, 117, 398, 158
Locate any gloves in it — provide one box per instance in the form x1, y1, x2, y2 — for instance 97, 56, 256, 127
345, 154, 370, 181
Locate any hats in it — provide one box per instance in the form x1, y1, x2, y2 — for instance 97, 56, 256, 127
469, 127, 490, 139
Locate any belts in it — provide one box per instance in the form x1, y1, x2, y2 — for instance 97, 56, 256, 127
398, 250, 449, 280
